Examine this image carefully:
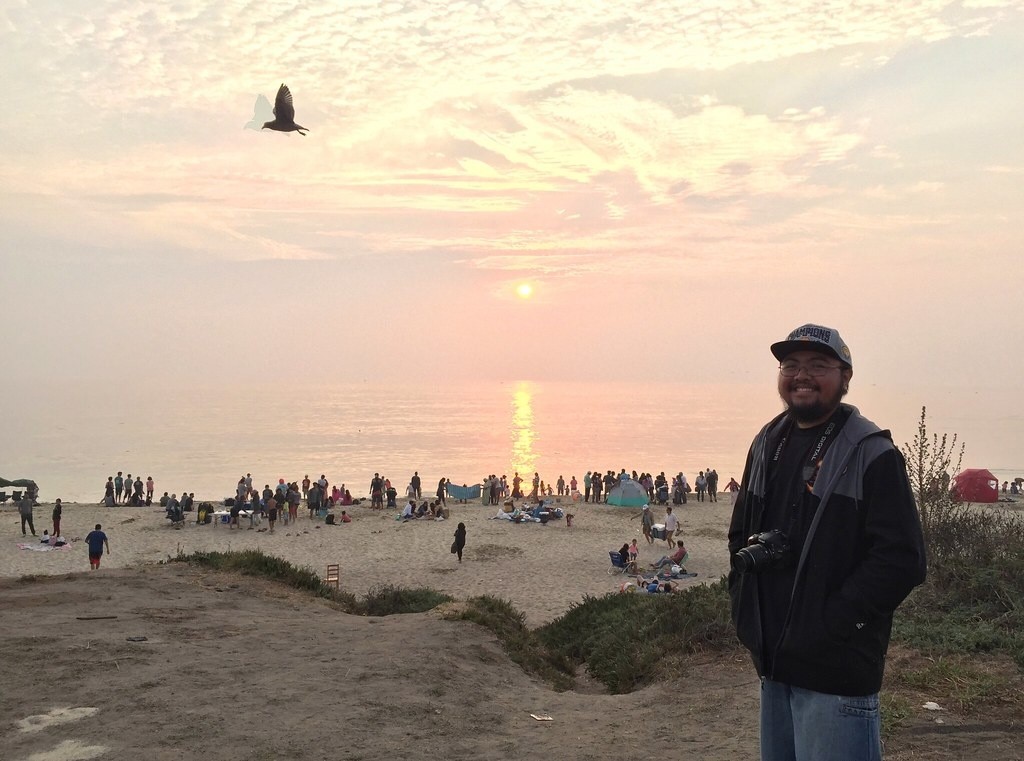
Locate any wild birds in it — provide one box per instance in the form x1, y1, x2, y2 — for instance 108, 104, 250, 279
261, 82, 311, 136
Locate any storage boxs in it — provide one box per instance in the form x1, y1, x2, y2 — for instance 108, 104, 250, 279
650, 524, 665, 539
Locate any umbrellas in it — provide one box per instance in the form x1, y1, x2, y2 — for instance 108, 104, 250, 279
0, 476, 33, 487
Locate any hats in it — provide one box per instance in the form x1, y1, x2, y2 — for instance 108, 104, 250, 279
770, 324, 852, 367
643, 504, 648, 510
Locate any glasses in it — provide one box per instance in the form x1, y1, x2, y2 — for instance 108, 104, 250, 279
780, 358, 844, 378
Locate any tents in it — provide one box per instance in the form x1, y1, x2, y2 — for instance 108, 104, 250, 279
607, 478, 649, 508
951, 469, 998, 503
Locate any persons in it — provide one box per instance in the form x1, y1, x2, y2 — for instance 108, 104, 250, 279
84, 524, 110, 570
728, 323, 927, 761
160, 471, 452, 534
930, 471, 1024, 494
450, 522, 467, 561
18, 480, 61, 543
98, 471, 154, 507
462, 468, 740, 518
618, 504, 687, 594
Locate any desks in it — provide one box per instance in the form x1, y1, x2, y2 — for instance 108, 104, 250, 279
242, 501, 284, 523
213, 511, 255, 528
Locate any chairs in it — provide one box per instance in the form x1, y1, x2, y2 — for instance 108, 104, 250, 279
636, 575, 681, 591
659, 553, 689, 573
323, 564, 339, 589
607, 550, 636, 574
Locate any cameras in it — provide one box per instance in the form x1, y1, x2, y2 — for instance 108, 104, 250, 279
734, 529, 795, 574
